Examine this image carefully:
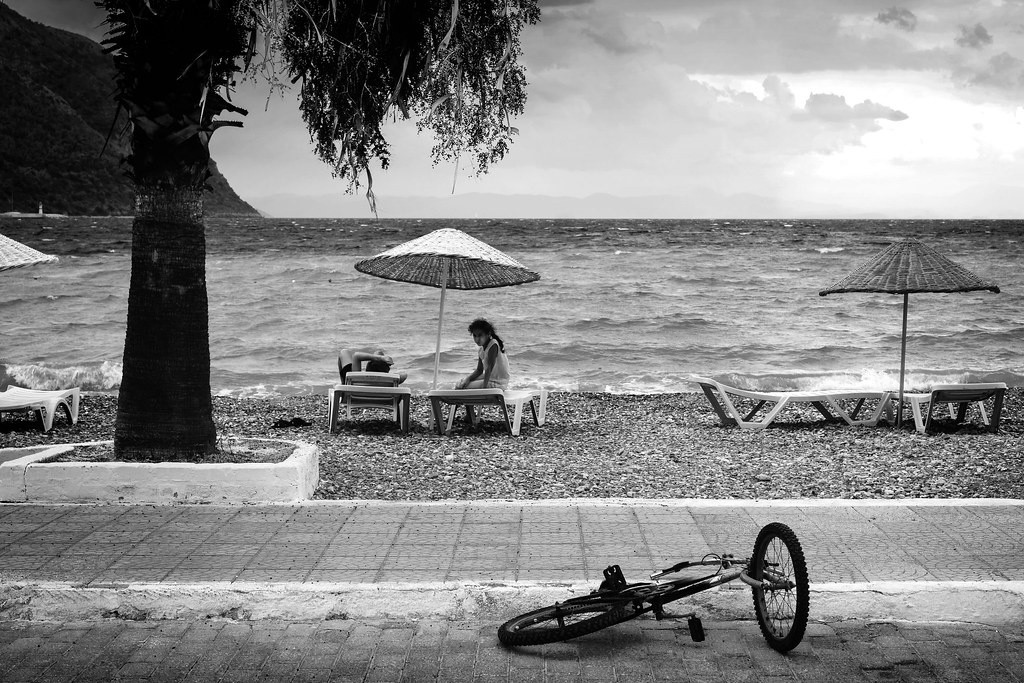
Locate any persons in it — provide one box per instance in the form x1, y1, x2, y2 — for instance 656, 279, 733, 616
445, 317, 510, 421
337, 347, 407, 384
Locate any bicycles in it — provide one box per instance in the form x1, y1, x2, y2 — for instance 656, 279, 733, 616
498, 523, 810, 651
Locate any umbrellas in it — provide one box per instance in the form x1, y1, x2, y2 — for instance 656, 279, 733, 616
0, 234, 60, 272
816, 232, 1000, 428
355, 228, 541, 430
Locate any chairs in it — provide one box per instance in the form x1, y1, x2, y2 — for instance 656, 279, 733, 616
883, 382, 1006, 434
0, 385, 81, 435
427, 388, 548, 438
327, 371, 411, 436
687, 375, 893, 430
